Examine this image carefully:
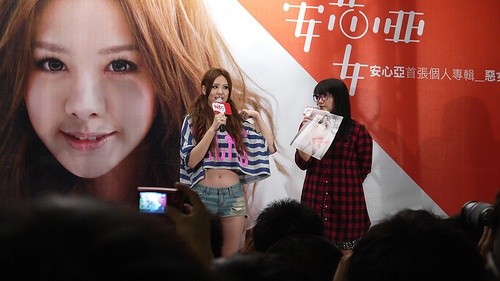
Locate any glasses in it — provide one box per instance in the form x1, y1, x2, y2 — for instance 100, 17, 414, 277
313, 93, 334, 102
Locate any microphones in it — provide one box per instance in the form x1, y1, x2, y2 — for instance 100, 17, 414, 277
212, 97, 232, 132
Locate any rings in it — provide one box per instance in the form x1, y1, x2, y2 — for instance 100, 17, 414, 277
221, 118, 223, 121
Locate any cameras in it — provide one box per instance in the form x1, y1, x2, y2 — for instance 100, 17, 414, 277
136, 186, 181, 216
462, 200, 498, 232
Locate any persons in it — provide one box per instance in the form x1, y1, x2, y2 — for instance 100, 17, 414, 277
0, 181, 500, 281
294, 78, 373, 255
0, 0, 292, 261
179, 67, 278, 260
